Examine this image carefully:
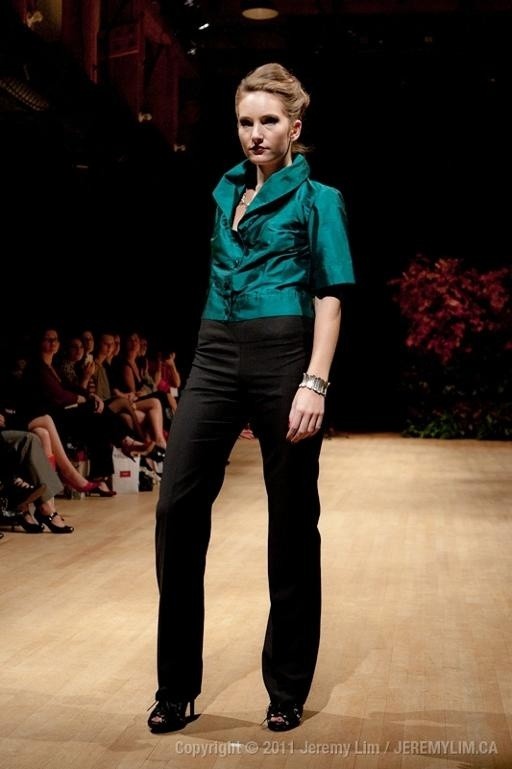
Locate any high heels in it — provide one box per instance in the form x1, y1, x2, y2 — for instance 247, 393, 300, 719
145, 681, 201, 732
1, 442, 174, 539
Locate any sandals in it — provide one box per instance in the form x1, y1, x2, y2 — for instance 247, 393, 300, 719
265, 692, 304, 735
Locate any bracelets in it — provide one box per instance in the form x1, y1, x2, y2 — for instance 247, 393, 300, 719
298, 372, 331, 397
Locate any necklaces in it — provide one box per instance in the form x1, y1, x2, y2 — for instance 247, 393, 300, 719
241, 186, 258, 206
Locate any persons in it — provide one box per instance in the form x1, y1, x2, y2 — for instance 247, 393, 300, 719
0, 323, 190, 538
138, 56, 357, 734
